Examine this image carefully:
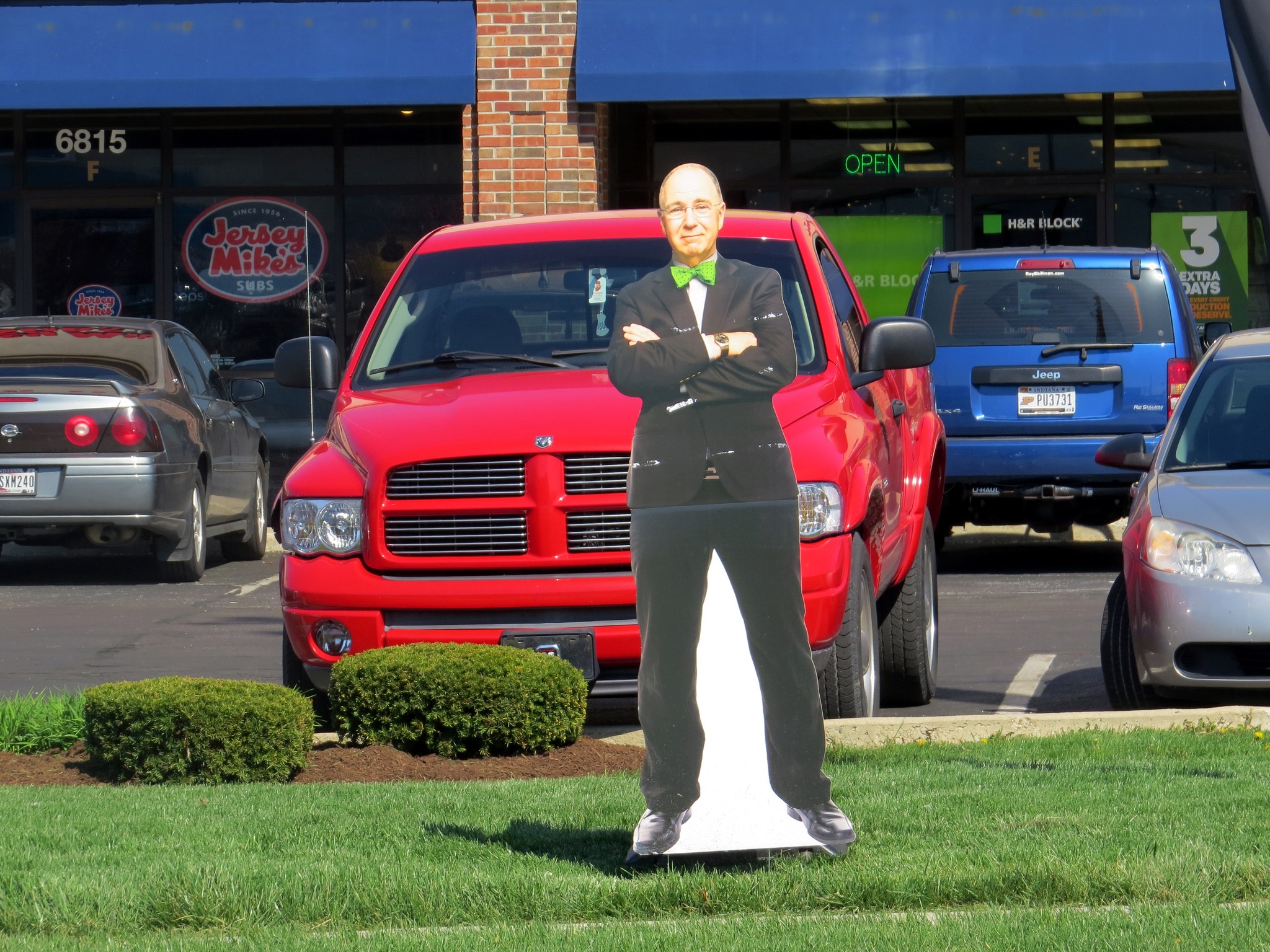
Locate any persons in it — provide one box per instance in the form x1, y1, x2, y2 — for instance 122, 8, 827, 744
605, 161, 858, 858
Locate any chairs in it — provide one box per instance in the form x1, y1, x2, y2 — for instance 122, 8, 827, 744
1230, 382, 1270, 462
449, 306, 523, 355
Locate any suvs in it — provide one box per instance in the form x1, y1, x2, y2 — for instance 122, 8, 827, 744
268, 206, 949, 727
897, 244, 1238, 545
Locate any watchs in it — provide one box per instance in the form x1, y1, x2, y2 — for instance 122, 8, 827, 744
715, 332, 729, 358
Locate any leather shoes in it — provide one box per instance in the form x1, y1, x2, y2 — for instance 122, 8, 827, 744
787, 798, 856, 845
633, 806, 692, 855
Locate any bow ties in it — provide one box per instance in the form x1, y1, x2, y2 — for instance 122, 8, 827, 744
671, 260, 716, 289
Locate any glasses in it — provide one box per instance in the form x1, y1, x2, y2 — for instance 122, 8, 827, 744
660, 203, 721, 219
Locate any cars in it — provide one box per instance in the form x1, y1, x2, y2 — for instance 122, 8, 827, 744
215, 359, 344, 526
1, 314, 270, 583
1093, 327, 1270, 719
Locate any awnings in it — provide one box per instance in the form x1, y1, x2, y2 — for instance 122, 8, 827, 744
0, 1, 480, 106
576, 0, 1236, 104
